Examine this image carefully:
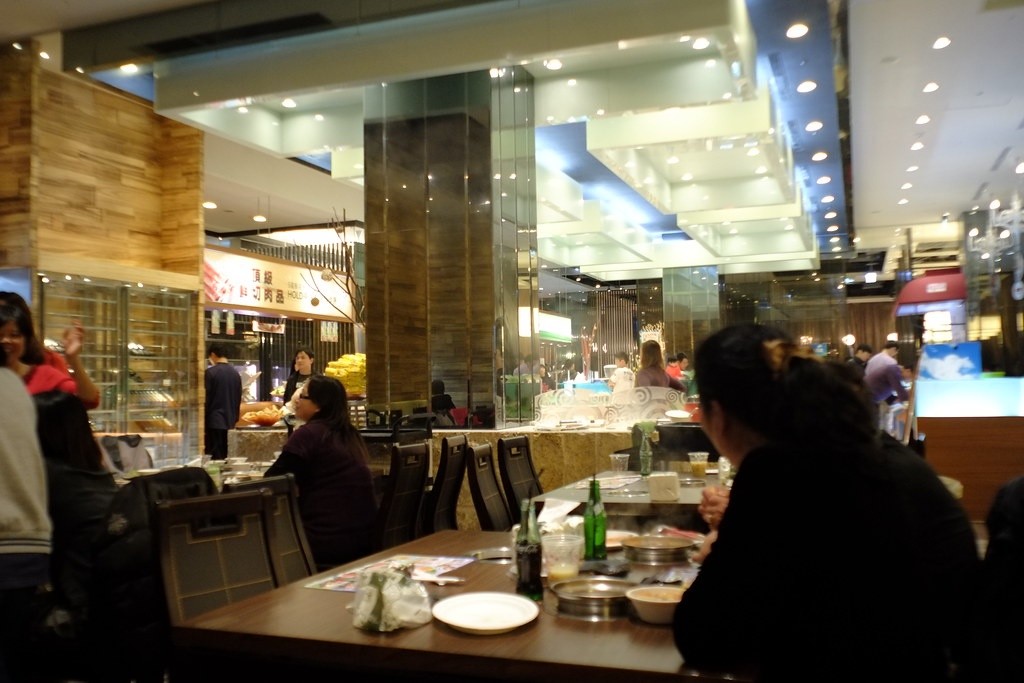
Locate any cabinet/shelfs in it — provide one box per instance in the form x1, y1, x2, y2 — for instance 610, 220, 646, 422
282, 413, 436, 491
37, 274, 191, 471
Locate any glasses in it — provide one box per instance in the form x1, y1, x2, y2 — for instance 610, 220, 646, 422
299, 389, 310, 400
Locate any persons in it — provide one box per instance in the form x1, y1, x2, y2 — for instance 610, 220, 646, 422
263, 375, 379, 567
282, 347, 317, 437
673, 321, 982, 683
635, 340, 686, 391
513, 354, 532, 376
865, 364, 916, 402
540, 359, 577, 392
667, 353, 688, 377
205, 343, 242, 459
864, 341, 899, 377
0, 290, 118, 683
608, 351, 634, 391
848, 344, 872, 369
432, 380, 456, 412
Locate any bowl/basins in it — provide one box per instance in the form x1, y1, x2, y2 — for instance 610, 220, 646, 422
209, 456, 250, 474
625, 585, 688, 623
665, 409, 693, 422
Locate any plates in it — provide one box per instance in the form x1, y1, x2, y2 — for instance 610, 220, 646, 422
432, 591, 540, 635
250, 417, 279, 426
680, 479, 705, 487
548, 574, 639, 615
604, 530, 639, 551
620, 538, 700, 563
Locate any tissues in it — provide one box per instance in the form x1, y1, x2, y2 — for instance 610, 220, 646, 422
511, 498, 585, 542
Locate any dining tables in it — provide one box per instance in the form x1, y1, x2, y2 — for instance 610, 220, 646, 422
167, 470, 756, 682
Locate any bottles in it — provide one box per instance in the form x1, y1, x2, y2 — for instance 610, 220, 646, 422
514, 498, 545, 600
584, 480, 608, 561
638, 433, 653, 475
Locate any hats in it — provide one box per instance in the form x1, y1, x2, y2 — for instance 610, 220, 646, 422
614, 352, 629, 363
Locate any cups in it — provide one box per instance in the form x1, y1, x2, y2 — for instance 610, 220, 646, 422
609, 453, 630, 475
539, 535, 584, 592
688, 452, 730, 479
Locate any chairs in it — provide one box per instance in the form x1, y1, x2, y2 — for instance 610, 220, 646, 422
147, 433, 543, 683
